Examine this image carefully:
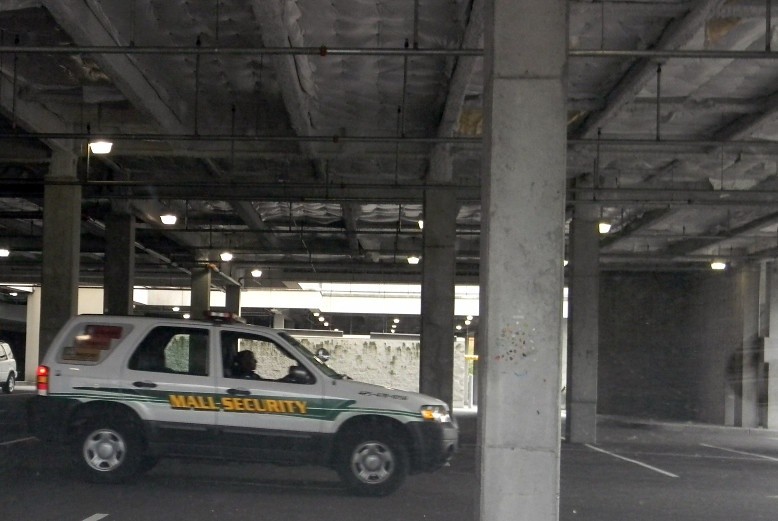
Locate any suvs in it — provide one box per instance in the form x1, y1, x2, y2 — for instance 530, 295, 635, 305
0, 340, 18, 394
24, 312, 459, 498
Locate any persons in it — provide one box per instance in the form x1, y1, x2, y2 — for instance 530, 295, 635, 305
232, 349, 262, 380
